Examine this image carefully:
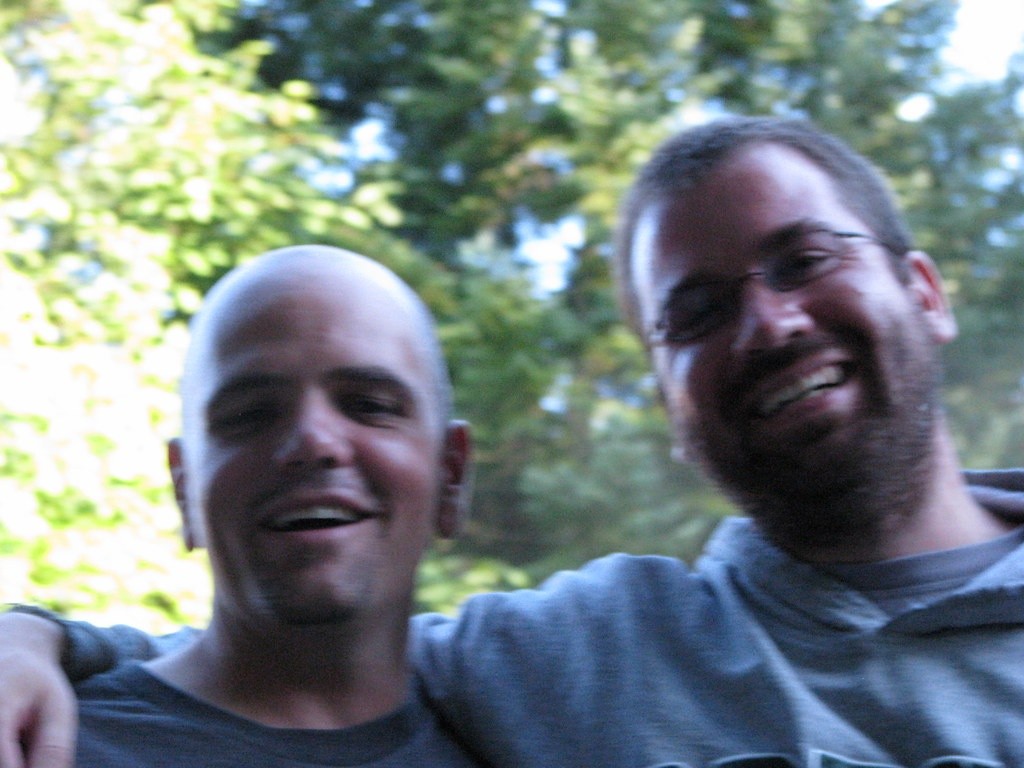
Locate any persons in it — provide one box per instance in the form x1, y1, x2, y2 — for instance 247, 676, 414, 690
77, 243, 480, 768
1, 116, 1022, 768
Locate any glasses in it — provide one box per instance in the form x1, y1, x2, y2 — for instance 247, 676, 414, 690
640, 227, 909, 343
